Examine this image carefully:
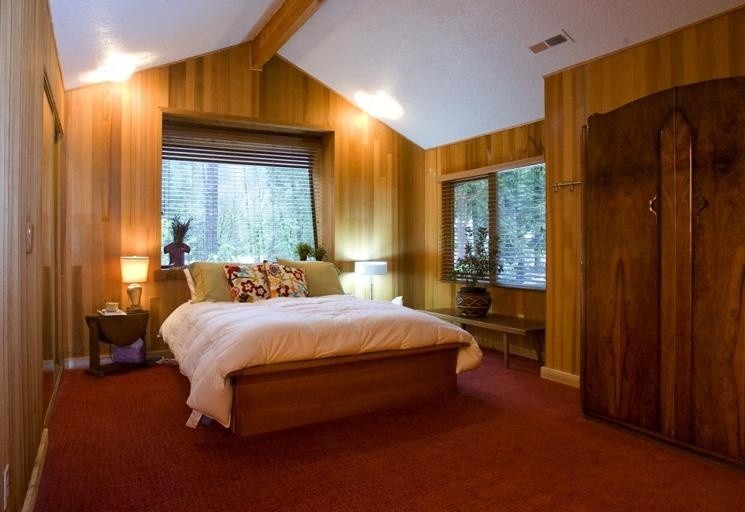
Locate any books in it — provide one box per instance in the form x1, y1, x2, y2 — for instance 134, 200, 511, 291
96, 308, 128, 316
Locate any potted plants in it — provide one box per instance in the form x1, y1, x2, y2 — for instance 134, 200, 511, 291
294, 242, 312, 261
163, 213, 195, 265
448, 222, 505, 320
311, 248, 328, 261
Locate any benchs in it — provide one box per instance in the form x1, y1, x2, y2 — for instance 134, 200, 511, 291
420, 304, 545, 370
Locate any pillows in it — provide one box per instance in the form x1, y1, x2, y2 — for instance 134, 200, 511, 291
275, 255, 347, 296
184, 267, 195, 301
264, 259, 309, 299
224, 263, 270, 303
189, 260, 232, 303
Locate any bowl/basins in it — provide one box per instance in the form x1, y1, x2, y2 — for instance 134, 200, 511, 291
105, 302, 119, 312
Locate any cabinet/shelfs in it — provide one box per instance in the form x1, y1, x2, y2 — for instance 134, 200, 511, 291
580, 72, 745, 475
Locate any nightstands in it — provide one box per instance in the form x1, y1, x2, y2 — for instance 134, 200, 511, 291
85, 309, 152, 378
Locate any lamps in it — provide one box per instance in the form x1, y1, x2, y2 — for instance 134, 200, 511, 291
354, 260, 387, 300
120, 255, 150, 313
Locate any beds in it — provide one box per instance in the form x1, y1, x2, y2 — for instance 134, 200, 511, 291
153, 256, 483, 440
